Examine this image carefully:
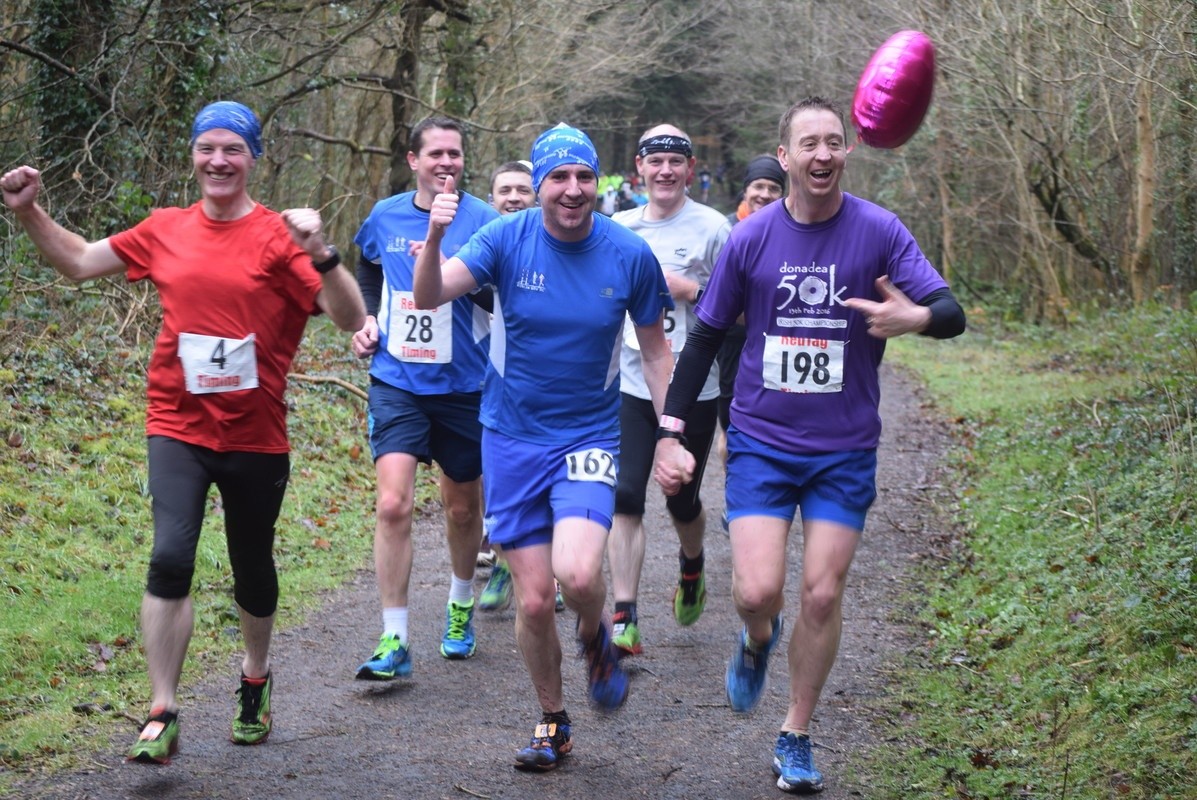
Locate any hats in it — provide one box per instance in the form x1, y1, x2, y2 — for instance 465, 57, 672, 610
530, 121, 599, 195
190, 101, 263, 158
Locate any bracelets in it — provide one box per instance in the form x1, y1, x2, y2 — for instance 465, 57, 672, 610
656, 426, 684, 440
311, 244, 342, 273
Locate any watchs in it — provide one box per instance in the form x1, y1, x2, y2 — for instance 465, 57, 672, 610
696, 285, 707, 302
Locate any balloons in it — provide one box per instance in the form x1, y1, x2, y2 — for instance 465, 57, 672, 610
853, 30, 938, 150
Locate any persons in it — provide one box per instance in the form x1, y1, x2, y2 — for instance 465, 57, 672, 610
339, 120, 503, 680
460, 161, 576, 613
709, 154, 781, 539
1, 101, 368, 766
590, 124, 738, 654
413, 117, 677, 769
648, 98, 970, 794
594, 170, 648, 220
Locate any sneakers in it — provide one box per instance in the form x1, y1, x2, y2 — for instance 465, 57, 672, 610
609, 613, 643, 656
673, 547, 708, 626
479, 556, 513, 612
571, 614, 629, 711
515, 715, 573, 768
770, 731, 825, 792
354, 630, 412, 680
476, 518, 498, 568
128, 704, 180, 765
725, 608, 782, 713
231, 666, 273, 744
551, 575, 565, 609
440, 599, 475, 659
